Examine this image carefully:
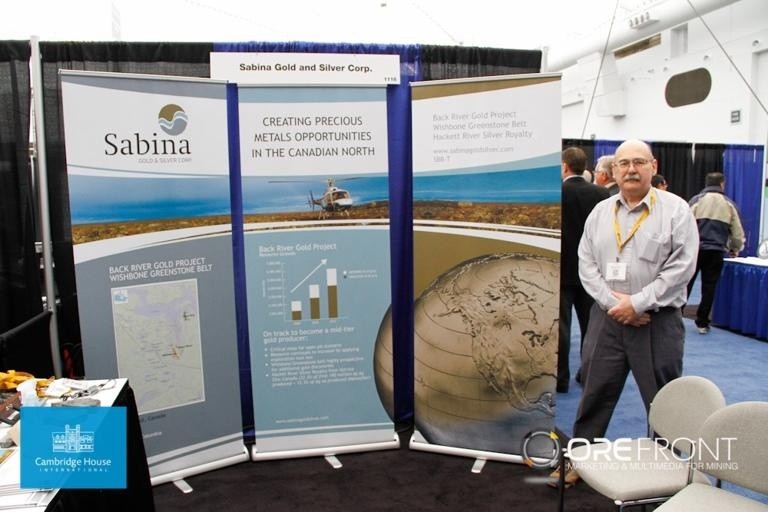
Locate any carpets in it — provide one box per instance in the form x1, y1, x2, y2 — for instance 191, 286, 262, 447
147, 417, 671, 512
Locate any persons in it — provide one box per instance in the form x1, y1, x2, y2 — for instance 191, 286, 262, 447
596, 155, 620, 196
547, 141, 700, 490
556, 145, 609, 394
681, 173, 746, 334
582, 169, 592, 183
651, 175, 668, 192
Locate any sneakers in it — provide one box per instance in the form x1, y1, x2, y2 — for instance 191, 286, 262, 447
698, 327, 708, 334
544, 463, 579, 489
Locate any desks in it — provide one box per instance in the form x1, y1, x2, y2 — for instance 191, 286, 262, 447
710, 254, 768, 342
0, 372, 155, 511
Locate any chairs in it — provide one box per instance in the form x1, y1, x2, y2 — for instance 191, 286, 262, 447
655, 400, 768, 512
558, 375, 725, 511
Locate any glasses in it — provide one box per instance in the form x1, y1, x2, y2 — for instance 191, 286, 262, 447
612, 158, 652, 170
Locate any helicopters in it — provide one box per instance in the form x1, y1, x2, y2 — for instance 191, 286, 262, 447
269, 177, 362, 220
